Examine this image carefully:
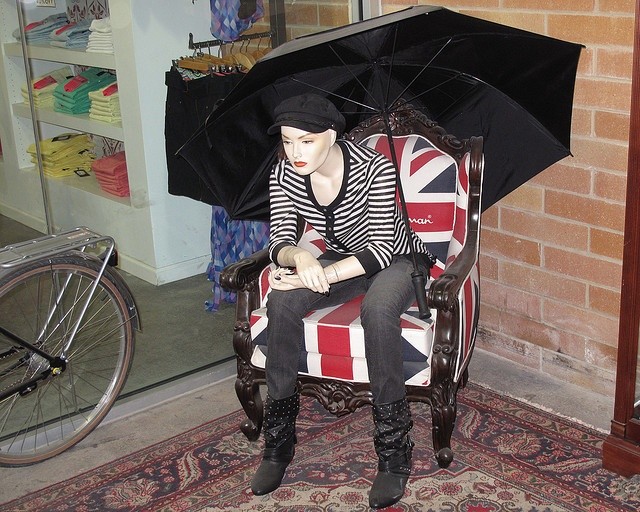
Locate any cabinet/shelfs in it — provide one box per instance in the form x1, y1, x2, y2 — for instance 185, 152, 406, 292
0, 2, 223, 287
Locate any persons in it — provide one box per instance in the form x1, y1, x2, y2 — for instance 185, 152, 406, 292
249, 92, 438, 507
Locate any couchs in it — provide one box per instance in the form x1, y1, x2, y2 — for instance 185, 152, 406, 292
220, 99, 484, 468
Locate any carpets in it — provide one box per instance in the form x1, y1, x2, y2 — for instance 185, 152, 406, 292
0, 384, 640, 512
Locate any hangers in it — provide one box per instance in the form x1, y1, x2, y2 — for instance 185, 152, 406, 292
173, 31, 281, 77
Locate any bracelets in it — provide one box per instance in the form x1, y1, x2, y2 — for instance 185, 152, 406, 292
331, 262, 343, 282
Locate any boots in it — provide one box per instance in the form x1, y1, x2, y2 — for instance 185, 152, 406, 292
251, 386, 299, 495
368, 396, 414, 508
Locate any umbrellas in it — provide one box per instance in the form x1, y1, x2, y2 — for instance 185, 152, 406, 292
176, 4, 586, 221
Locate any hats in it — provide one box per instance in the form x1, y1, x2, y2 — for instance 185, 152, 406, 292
267, 92, 346, 135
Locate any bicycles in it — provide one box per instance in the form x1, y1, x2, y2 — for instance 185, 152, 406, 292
0, 226, 143, 467
